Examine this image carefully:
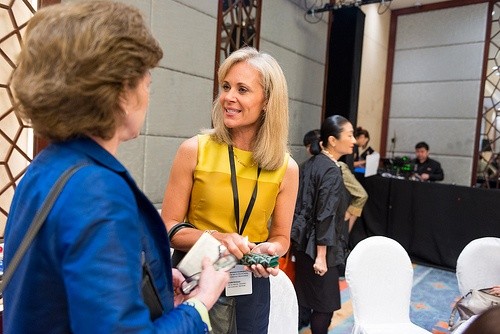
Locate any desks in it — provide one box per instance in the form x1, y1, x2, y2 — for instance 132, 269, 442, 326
348, 173, 500, 270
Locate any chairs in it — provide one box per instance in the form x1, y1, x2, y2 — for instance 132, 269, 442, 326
345, 236, 433, 334
456, 237, 500, 296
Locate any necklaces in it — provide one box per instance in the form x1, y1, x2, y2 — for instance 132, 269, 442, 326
233, 153, 258, 168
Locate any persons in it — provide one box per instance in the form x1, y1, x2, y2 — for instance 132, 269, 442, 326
289, 115, 356, 334
2, 0, 230, 334
159, 45, 300, 334
409, 142, 444, 181
353, 131, 374, 168
303, 129, 368, 265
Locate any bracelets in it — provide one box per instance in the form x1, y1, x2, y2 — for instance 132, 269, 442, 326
204, 230, 217, 235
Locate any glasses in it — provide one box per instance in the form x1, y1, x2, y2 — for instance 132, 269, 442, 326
175, 253, 239, 294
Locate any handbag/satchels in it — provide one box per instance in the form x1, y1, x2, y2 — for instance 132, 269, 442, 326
202, 297, 237, 334
